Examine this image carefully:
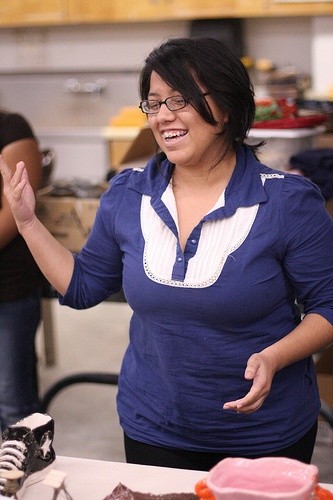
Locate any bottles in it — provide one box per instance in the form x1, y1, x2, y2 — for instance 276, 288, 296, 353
255, 58, 273, 84
240, 56, 255, 85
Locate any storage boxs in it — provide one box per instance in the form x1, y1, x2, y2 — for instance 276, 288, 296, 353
36, 124, 111, 184
106, 126, 141, 172
238, 125, 326, 170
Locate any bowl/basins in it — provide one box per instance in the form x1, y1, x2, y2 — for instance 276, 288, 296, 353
206, 457, 319, 500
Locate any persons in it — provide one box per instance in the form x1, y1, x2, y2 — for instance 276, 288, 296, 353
0, 38, 333, 472
0, 108, 43, 443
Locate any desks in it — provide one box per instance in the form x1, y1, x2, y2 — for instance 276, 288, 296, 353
21, 456, 333, 500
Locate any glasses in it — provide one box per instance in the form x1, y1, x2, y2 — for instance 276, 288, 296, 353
139, 92, 211, 114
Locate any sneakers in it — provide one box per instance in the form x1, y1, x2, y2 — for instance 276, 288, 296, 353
0, 410, 56, 498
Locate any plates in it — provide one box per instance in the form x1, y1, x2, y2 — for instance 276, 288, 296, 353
195, 477, 333, 500
